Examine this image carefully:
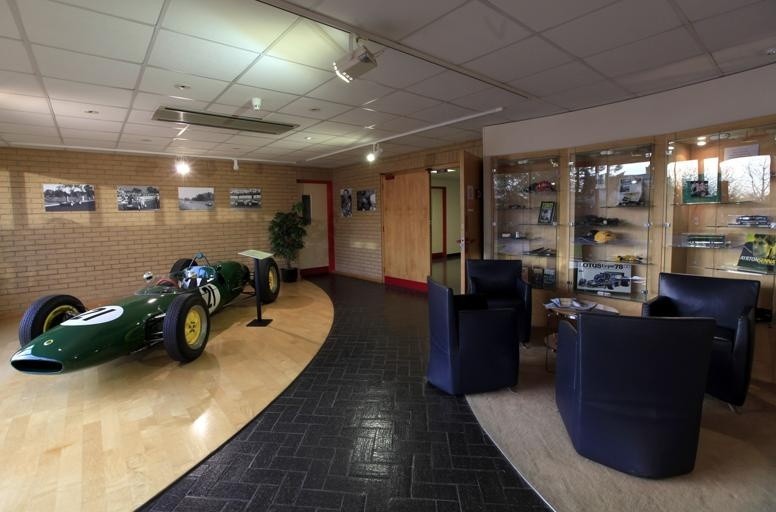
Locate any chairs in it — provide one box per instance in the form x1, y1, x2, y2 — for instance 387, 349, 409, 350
465, 257, 535, 350
556, 310, 718, 479
422, 276, 521, 393
641, 272, 761, 416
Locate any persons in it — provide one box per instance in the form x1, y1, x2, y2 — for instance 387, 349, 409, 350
740, 234, 776, 270
341, 189, 351, 207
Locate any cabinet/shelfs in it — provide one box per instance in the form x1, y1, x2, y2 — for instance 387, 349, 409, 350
656, 118, 775, 322
568, 138, 655, 302
490, 148, 566, 288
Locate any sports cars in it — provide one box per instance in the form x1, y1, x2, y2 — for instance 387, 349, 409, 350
119, 194, 147, 209
579, 270, 631, 290
205, 201, 212, 206
59, 198, 82, 207
9, 252, 281, 375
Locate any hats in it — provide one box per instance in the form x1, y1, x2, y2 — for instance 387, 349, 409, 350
577, 229, 618, 244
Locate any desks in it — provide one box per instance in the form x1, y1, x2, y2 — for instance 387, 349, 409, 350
545, 298, 618, 374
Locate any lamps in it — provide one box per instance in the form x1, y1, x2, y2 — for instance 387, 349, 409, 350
332, 31, 379, 86
172, 155, 241, 174
365, 142, 384, 164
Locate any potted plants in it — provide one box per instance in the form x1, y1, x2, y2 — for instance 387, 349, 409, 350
267, 211, 304, 284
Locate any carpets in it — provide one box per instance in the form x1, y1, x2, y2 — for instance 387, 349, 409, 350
463, 326, 775, 511
